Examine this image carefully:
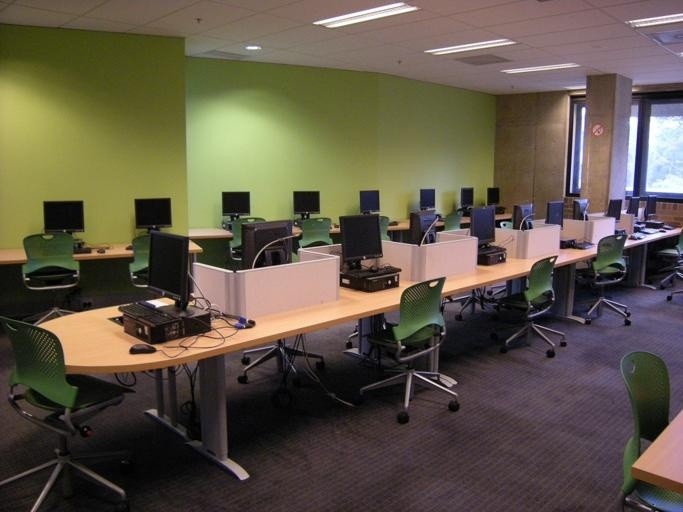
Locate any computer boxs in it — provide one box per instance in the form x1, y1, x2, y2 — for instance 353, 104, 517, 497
645, 220, 664, 228
560, 237, 575, 249
478, 252, 506, 266
340, 269, 400, 292
615, 228, 625, 236
123, 305, 211, 345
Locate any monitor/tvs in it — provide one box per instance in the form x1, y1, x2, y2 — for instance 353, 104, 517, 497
627, 197, 640, 215
606, 200, 622, 222
293, 190, 321, 219
487, 187, 500, 205
573, 199, 590, 220
147, 230, 195, 315
43, 200, 85, 233
547, 201, 564, 229
420, 188, 436, 209
360, 190, 380, 214
241, 220, 293, 268
340, 214, 384, 269
222, 191, 251, 216
134, 197, 172, 232
645, 194, 657, 216
461, 188, 474, 206
512, 202, 535, 230
409, 210, 437, 243
470, 205, 495, 243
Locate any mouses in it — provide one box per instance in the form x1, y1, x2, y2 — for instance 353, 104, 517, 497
369, 265, 379, 272
659, 229, 666, 232
129, 344, 156, 355
97, 247, 105, 253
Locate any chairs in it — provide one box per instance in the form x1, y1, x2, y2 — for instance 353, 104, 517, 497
22, 231, 80, 327
441, 287, 492, 321
298, 218, 333, 248
345, 319, 398, 350
379, 215, 390, 240
444, 213, 461, 230
128, 234, 152, 289
607, 350, 683, 511
229, 218, 266, 261
237, 252, 326, 385
0, 317, 127, 512
358, 276, 460, 425
487, 221, 513, 301
584, 232, 632, 326
499, 255, 566, 358
655, 228, 683, 302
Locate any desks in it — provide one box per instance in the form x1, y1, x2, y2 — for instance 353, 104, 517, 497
631, 409, 683, 495
36, 221, 683, 481
0, 238, 204, 276
292, 212, 513, 234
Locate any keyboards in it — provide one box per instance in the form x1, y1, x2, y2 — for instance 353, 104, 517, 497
640, 227, 659, 233
478, 245, 507, 255
347, 266, 402, 279
630, 232, 644, 239
73, 247, 92, 254
663, 223, 675, 230
575, 242, 593, 249
118, 299, 180, 328
